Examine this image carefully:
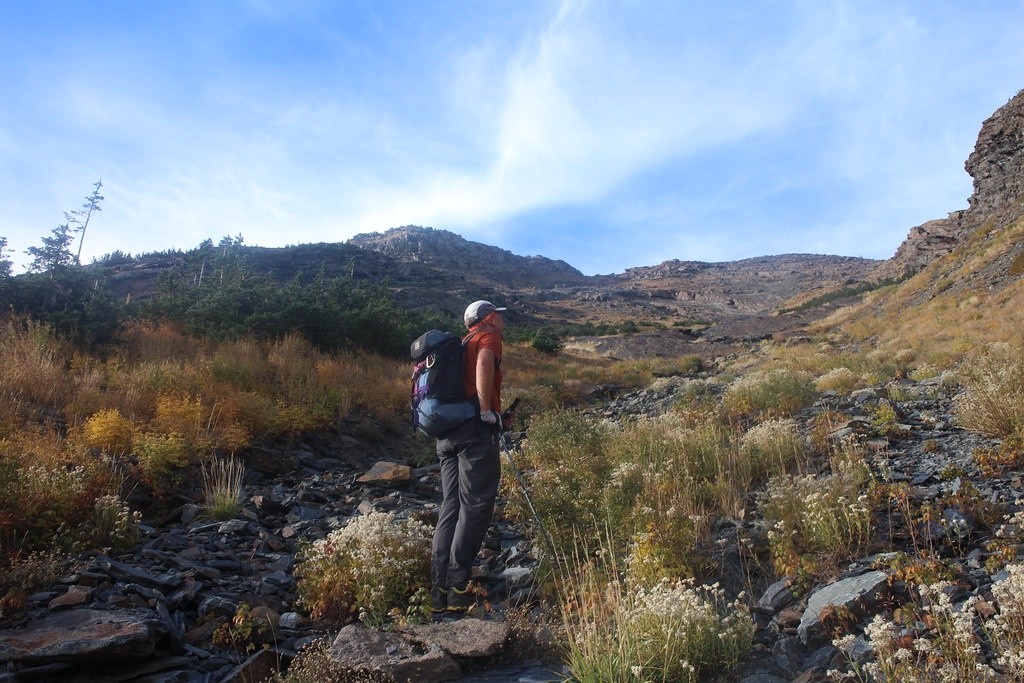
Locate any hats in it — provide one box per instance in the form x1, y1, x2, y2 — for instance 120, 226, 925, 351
463, 300, 506, 329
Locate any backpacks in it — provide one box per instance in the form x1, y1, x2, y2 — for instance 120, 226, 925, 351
410, 328, 487, 439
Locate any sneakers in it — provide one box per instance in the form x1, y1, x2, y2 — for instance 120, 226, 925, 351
430, 586, 448, 612
446, 582, 491, 612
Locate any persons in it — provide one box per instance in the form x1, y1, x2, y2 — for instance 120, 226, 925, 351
411, 299, 509, 612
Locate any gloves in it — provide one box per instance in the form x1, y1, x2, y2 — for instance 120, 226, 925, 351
480, 409, 497, 425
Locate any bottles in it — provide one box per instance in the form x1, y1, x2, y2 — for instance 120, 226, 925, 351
502, 398, 521, 431
